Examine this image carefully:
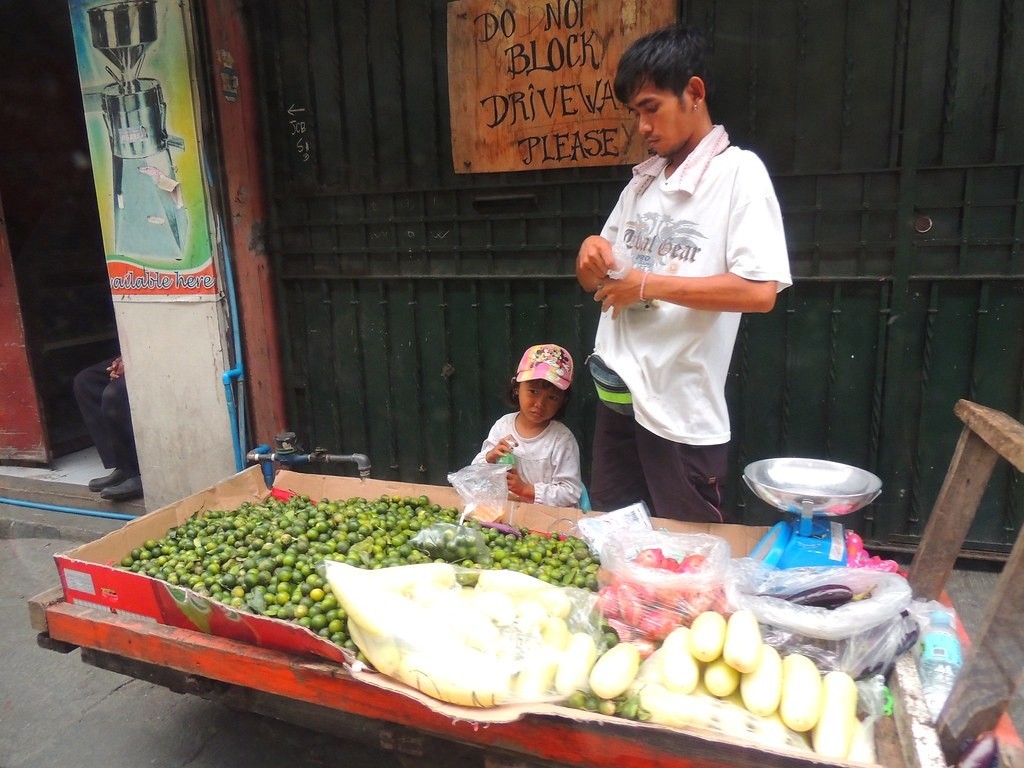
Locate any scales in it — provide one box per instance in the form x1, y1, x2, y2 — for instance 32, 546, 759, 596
742, 458, 883, 570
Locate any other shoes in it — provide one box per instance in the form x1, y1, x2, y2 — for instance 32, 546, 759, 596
101, 474, 144, 500
89, 468, 139, 492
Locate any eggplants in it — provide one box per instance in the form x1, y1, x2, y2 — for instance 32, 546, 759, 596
746, 584, 922, 682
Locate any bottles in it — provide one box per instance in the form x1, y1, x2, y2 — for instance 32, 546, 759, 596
496, 441, 516, 466
918, 612, 964, 724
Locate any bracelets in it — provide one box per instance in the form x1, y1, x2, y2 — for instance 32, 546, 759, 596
640, 270, 654, 304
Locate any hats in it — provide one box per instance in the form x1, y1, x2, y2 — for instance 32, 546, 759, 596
517, 344, 573, 391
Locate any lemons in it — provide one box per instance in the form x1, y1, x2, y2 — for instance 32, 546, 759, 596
114, 494, 641, 721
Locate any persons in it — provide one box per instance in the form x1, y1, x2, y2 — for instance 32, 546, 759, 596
73, 355, 143, 499
471, 343, 582, 508
575, 25, 793, 525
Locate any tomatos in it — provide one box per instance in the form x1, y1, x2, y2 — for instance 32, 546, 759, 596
597, 548, 727, 656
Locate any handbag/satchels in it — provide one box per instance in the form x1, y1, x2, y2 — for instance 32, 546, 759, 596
584, 354, 635, 418
